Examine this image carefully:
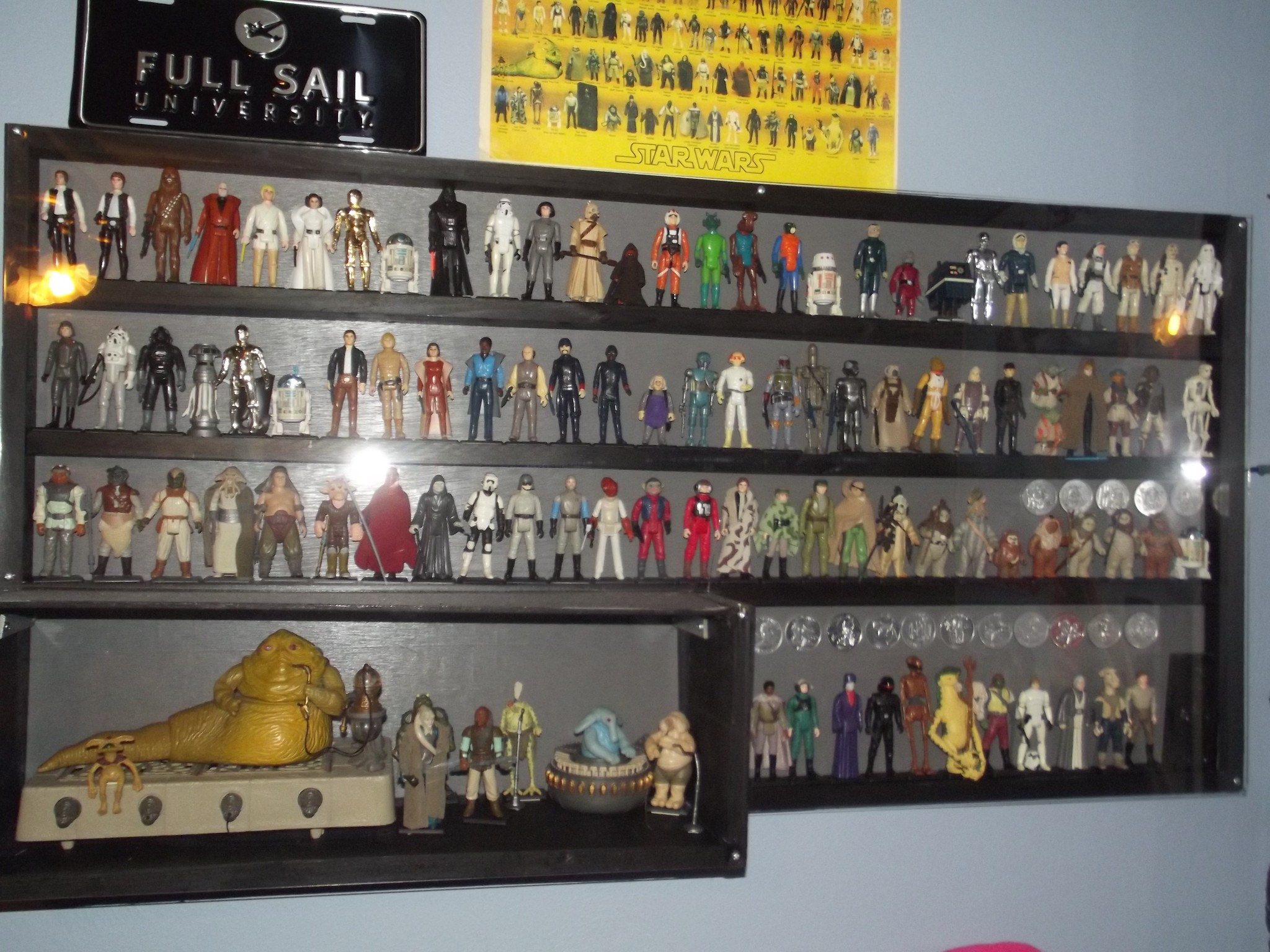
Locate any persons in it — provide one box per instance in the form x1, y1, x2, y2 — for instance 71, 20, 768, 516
326, 330, 368, 440
1044, 240, 1078, 328
853, 222, 887, 321
547, 475, 591, 582
490, 0, 893, 155
592, 345, 632, 448
214, 324, 270, 434
994, 363, 1027, 456
501, 346, 550, 443
32, 170, 1224, 834
415, 342, 455, 441
240, 184, 288, 289
717, 476, 759, 580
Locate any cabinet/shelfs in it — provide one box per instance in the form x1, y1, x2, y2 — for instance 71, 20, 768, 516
2, 121, 1253, 902
